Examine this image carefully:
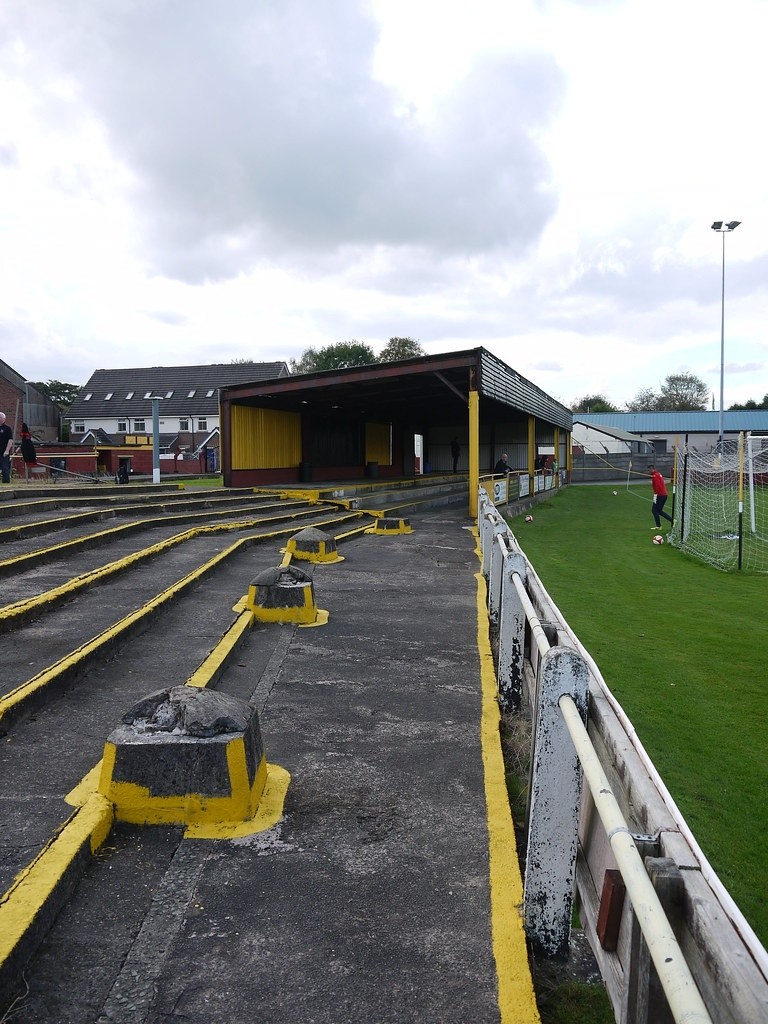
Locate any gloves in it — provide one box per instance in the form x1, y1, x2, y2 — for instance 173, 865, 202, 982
652, 494, 657, 504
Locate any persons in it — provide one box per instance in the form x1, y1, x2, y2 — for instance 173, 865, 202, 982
535, 453, 558, 473
493, 454, 517, 479
450, 436, 460, 471
646, 465, 676, 530
0, 412, 13, 483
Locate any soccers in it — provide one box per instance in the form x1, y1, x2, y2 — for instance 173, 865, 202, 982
525, 515, 534, 523
612, 490, 618, 496
652, 535, 664, 545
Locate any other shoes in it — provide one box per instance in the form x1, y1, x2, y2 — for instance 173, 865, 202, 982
672, 519, 677, 527
650, 526, 662, 531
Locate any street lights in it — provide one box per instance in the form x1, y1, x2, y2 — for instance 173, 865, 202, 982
711, 221, 741, 453
